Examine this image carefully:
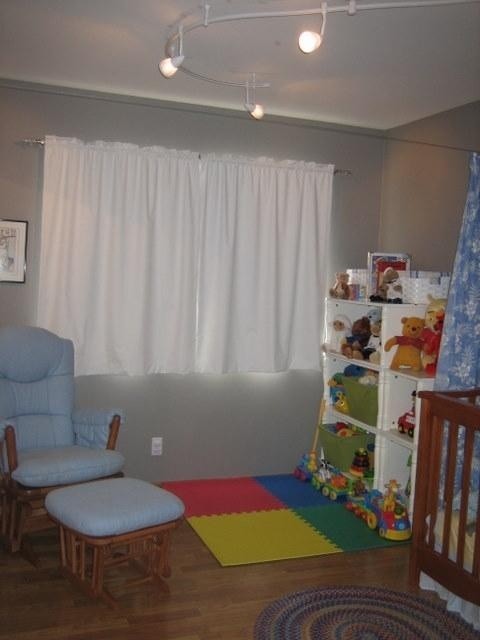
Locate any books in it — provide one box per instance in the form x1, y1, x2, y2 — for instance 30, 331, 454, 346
366, 250, 412, 299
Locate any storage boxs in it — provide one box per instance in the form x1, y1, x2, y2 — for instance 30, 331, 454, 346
317, 421, 376, 471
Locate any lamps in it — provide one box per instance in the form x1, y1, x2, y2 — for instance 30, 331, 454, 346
159, 1, 329, 119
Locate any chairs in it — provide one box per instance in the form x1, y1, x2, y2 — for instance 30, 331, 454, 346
0, 325, 170, 611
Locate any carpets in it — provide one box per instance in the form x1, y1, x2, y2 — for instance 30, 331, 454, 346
252, 584, 480, 640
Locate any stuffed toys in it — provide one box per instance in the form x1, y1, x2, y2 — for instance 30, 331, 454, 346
320, 266, 448, 377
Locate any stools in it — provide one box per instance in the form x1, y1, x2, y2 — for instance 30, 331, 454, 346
46, 476, 186, 593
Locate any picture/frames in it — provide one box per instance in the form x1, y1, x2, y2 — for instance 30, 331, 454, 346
0, 219, 28, 284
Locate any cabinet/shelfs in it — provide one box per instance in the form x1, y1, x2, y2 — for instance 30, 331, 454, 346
324, 296, 436, 533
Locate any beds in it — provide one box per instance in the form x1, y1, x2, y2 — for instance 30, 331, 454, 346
409, 387, 479, 606
